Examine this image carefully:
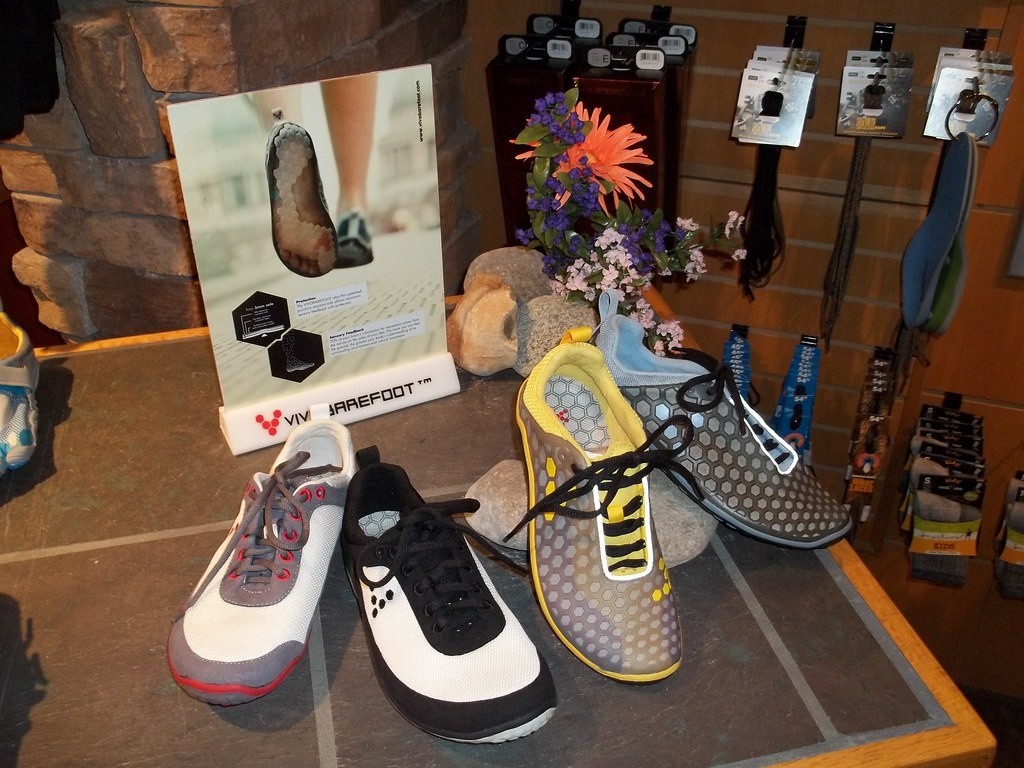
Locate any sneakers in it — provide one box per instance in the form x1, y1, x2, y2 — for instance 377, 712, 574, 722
167, 418, 358, 705
515, 327, 685, 686
342, 445, 558, 744
267, 118, 375, 279
589, 288, 852, 552
0, 311, 38, 477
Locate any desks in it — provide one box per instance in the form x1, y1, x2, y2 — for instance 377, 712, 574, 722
0, 279, 998, 768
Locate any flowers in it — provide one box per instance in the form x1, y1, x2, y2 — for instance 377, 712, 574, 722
508, 86, 749, 359
